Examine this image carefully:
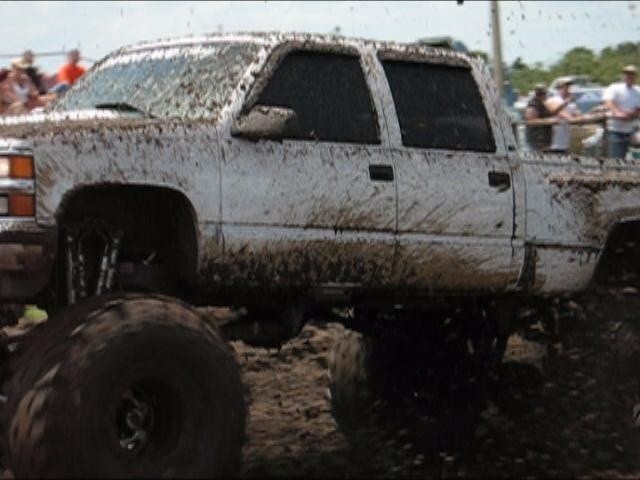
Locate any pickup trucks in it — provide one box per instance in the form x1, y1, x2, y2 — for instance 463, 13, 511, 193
0, 28, 638, 351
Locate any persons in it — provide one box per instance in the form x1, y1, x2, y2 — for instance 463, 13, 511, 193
53, 48, 86, 85
543, 76, 590, 157
0, 48, 58, 116
523, 86, 554, 150
600, 63, 640, 163
